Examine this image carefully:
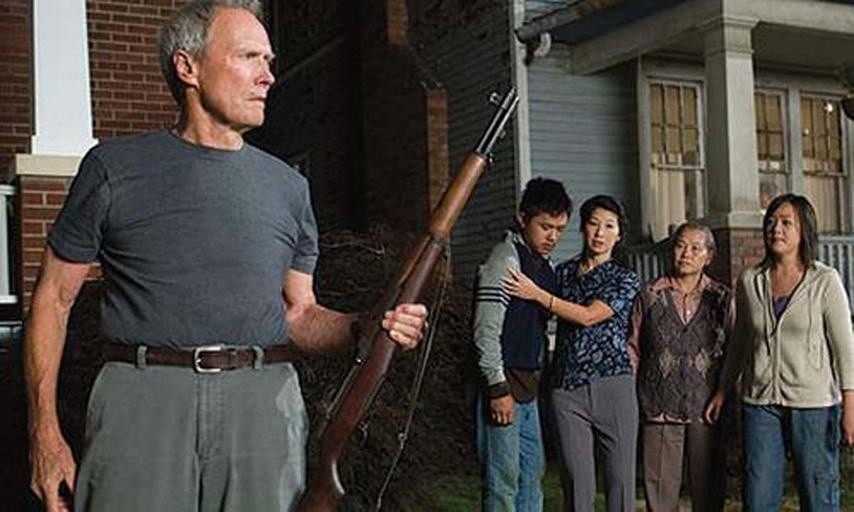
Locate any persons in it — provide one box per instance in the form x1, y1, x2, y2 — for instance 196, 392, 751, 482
469, 174, 577, 512
623, 224, 736, 512
497, 195, 645, 512
13, 0, 429, 512
700, 193, 854, 512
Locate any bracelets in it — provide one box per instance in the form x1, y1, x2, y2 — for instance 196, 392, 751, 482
546, 294, 557, 312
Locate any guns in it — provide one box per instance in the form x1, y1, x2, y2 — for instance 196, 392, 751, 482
287, 83, 520, 512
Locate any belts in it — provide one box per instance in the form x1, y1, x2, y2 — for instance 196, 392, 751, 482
108, 344, 293, 373
